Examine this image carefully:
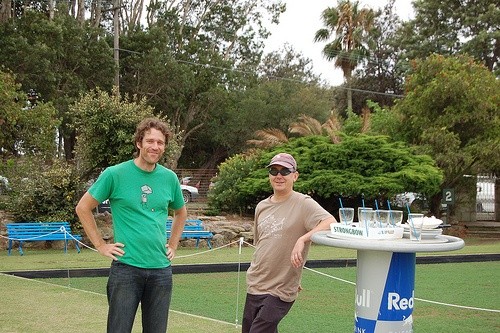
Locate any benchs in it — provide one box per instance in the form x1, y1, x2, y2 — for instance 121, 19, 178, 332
4, 221, 82, 256
166, 219, 214, 249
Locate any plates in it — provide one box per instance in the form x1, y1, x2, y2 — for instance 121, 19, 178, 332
403, 228, 443, 239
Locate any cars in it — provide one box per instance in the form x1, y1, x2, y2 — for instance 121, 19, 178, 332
102, 184, 200, 205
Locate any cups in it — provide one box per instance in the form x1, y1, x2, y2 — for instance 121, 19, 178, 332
357, 207, 403, 228
408, 214, 423, 240
339, 208, 354, 227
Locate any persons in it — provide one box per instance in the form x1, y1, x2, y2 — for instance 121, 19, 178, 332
75, 121, 188, 333
242, 152, 338, 333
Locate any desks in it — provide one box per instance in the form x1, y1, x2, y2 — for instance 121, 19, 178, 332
310, 230, 466, 333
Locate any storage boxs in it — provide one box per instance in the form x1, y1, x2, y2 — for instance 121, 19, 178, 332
330, 223, 404, 240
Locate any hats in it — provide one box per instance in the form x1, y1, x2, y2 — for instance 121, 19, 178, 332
266, 153, 296, 169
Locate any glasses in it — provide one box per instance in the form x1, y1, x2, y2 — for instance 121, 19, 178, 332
269, 167, 295, 176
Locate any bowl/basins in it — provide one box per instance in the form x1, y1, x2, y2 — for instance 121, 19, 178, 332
407, 218, 443, 229
